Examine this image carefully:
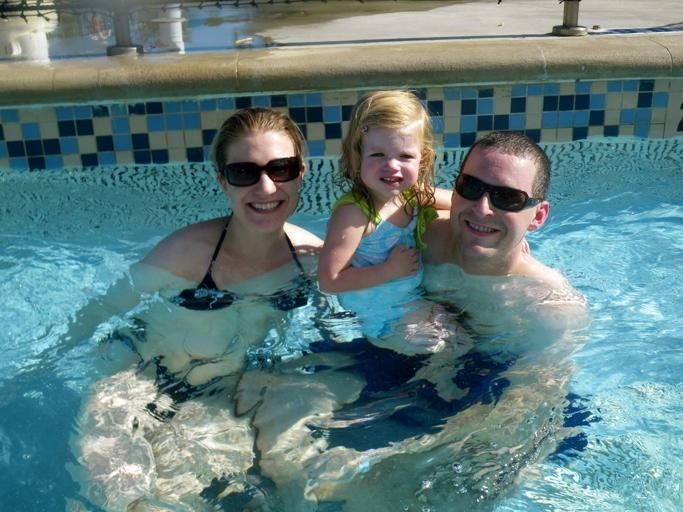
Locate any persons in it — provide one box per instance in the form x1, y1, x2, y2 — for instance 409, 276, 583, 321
252, 129, 594, 512
316, 87, 532, 404
0, 107, 325, 512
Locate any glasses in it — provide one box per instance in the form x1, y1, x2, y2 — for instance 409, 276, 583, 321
222, 157, 301, 186
455, 173, 541, 212
